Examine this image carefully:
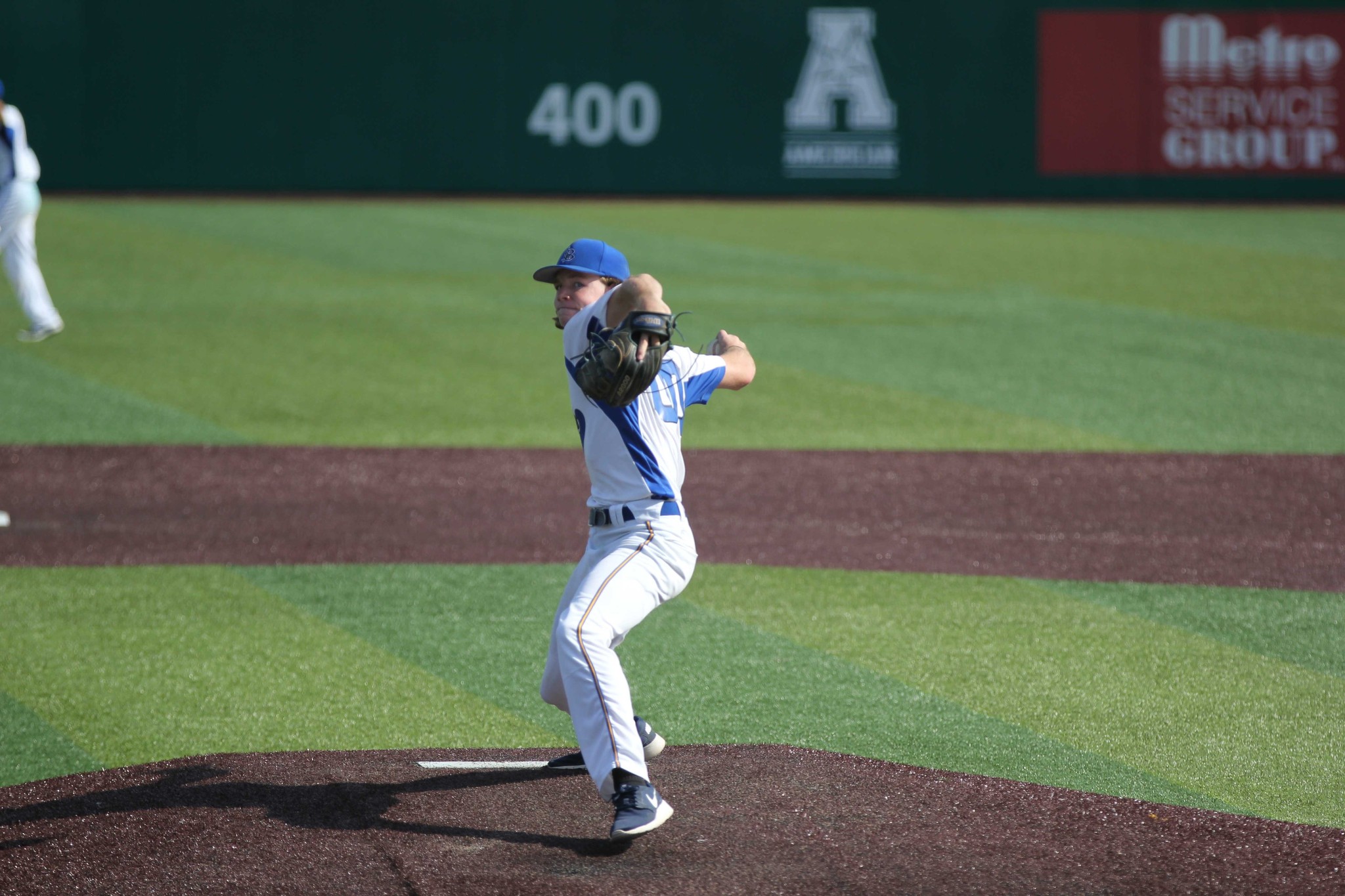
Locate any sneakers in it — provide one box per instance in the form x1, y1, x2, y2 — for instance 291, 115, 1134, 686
545, 715, 665, 772
606, 781, 674, 841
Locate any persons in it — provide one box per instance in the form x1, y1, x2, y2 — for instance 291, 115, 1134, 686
0, 77, 66, 344
532, 237, 756, 843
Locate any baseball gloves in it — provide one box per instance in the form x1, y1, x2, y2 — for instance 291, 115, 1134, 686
576, 314, 675, 408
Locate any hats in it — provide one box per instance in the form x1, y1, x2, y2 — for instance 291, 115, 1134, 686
532, 238, 632, 283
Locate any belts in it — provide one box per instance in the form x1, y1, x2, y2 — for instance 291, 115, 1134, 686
586, 501, 684, 529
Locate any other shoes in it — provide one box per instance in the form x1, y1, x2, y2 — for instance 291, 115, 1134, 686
15, 318, 66, 345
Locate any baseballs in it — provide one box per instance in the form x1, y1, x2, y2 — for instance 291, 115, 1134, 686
706, 338, 719, 355
